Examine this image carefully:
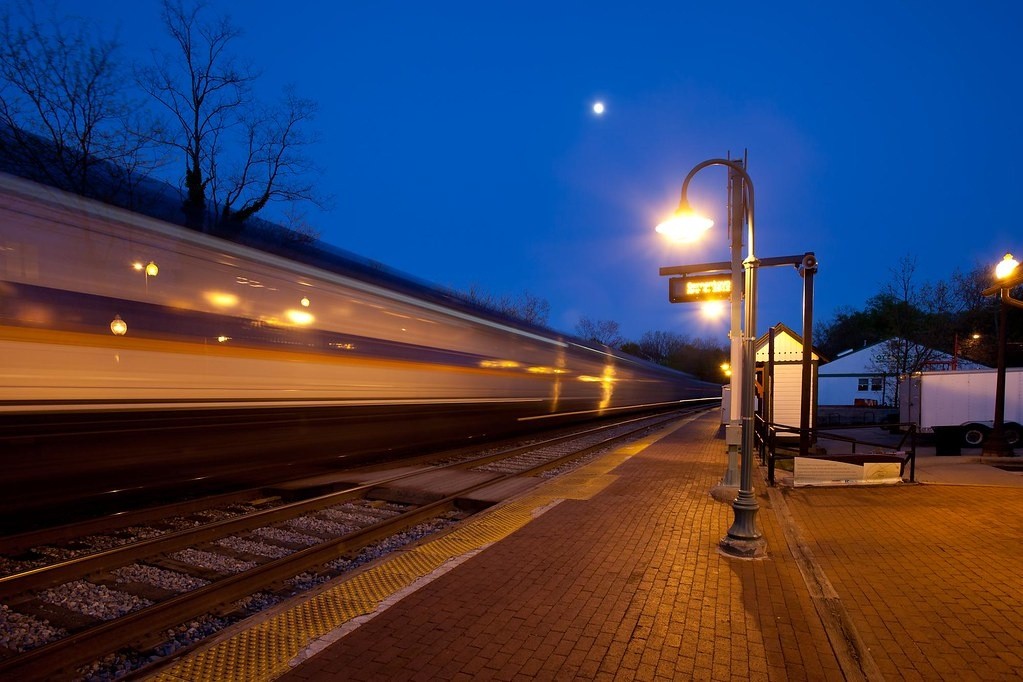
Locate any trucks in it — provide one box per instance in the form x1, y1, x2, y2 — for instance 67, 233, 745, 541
897, 365, 1023, 448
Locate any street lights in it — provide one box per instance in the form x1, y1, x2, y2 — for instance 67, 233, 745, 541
653, 156, 769, 557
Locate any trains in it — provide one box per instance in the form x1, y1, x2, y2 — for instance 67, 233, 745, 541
0, 168, 724, 501
